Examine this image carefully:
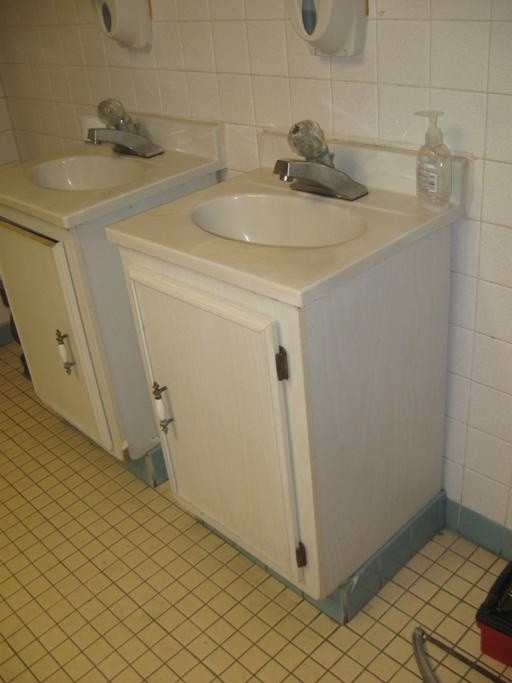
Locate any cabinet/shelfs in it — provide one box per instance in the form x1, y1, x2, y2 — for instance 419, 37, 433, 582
119, 225, 452, 627
0, 170, 219, 487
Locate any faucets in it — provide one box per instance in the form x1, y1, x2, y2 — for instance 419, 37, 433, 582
271, 119, 367, 201
83, 96, 166, 158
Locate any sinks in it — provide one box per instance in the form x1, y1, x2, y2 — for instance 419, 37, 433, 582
24, 155, 146, 191
192, 192, 367, 246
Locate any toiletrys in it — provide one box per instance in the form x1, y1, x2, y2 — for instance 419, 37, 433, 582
411, 109, 452, 211
